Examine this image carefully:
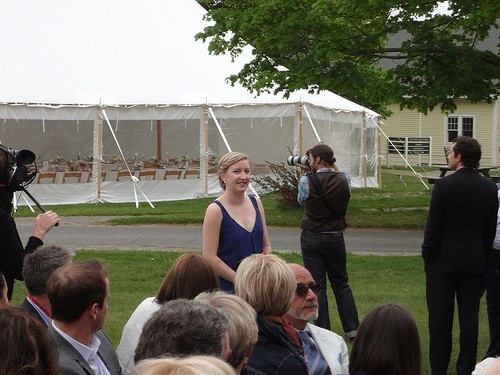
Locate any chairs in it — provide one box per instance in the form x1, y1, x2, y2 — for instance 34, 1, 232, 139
37, 172, 57, 184
183, 170, 200, 180
63, 160, 93, 172
138, 171, 156, 180
163, 170, 182, 180
87, 171, 106, 182
116, 171, 134, 181
62, 172, 82, 183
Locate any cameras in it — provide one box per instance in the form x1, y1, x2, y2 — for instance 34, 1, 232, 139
287, 156, 310, 167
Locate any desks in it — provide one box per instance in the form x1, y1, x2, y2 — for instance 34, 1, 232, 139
421, 165, 500, 191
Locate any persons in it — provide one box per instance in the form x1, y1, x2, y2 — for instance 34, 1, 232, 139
422, 136, 499, 375
202, 152, 271, 296
0, 246, 500, 375
0, 151, 60, 303
481, 147, 500, 361
297, 144, 362, 341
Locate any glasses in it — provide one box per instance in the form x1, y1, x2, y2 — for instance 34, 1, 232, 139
294, 283, 322, 299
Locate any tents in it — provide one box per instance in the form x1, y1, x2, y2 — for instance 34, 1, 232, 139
0, 0, 429, 212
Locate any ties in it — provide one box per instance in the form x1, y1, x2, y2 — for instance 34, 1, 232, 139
297, 329, 333, 375
